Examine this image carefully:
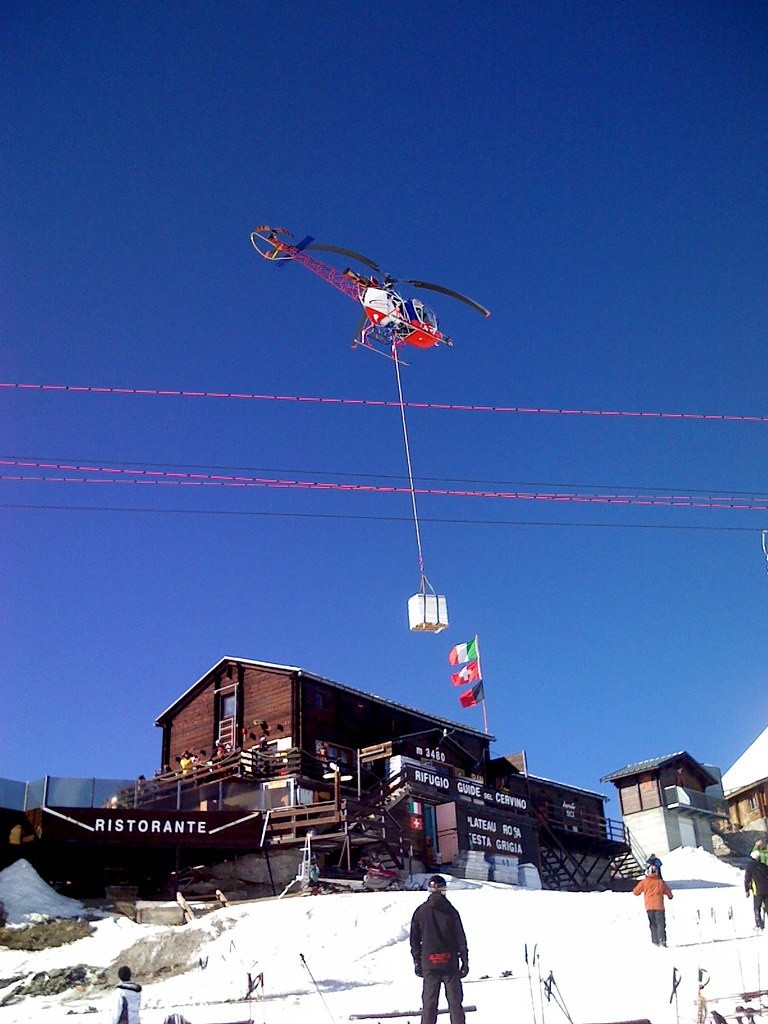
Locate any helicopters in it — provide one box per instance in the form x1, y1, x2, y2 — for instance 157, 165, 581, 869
248, 226, 490, 368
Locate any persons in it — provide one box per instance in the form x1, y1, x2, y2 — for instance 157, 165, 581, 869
749, 837, 768, 869
646, 854, 663, 882
318, 739, 331, 776
744, 851, 768, 930
111, 965, 142, 1024
128, 732, 276, 806
435, 850, 443, 873
410, 876, 470, 1024
633, 865, 673, 947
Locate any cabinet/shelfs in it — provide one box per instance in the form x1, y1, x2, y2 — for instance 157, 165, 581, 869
407, 593, 448, 633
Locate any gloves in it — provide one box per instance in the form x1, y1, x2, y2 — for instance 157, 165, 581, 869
414, 963, 423, 977
458, 963, 469, 978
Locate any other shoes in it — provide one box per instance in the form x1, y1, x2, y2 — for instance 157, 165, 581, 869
652, 939, 667, 947
753, 925, 767, 932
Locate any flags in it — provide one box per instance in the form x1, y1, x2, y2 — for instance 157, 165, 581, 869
449, 635, 481, 666
458, 679, 485, 709
450, 658, 482, 687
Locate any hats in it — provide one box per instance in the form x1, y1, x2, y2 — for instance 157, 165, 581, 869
749, 851, 762, 862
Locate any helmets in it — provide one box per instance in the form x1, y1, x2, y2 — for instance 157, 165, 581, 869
426, 874, 447, 896
